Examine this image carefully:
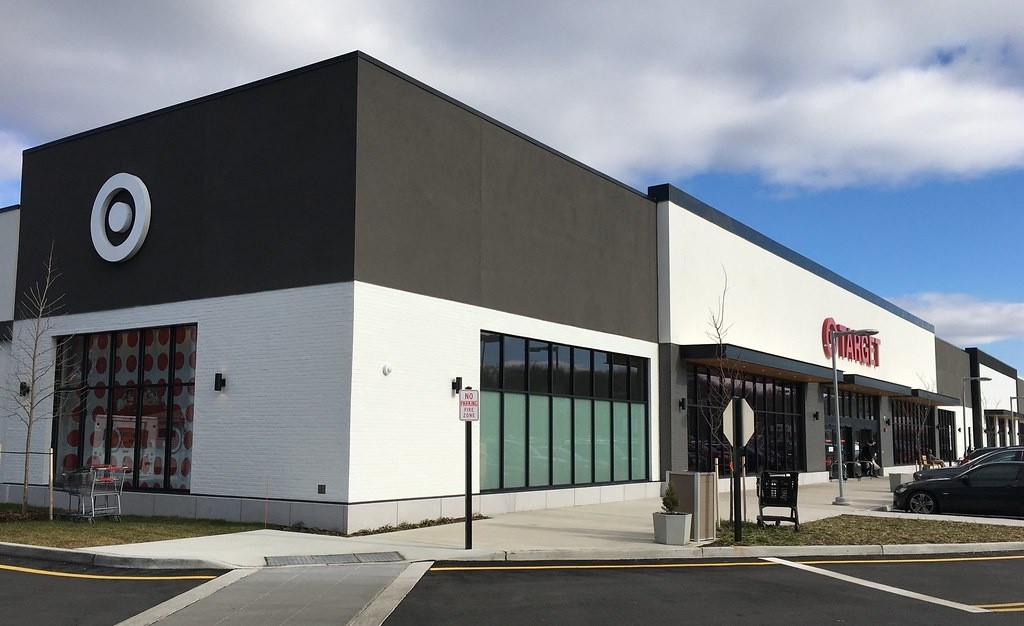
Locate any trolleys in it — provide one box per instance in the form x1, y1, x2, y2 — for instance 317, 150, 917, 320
55, 465, 132, 523
754, 471, 800, 533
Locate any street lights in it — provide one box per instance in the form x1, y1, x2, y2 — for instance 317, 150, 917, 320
831, 330, 879, 506
963, 377, 993, 458
1010, 396, 1024, 446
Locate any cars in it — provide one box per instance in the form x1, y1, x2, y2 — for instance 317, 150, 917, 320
892, 445, 1024, 517
485, 435, 642, 484
686, 421, 864, 478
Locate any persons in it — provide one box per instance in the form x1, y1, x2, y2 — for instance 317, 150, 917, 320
861, 439, 879, 479
967, 447, 971, 455
926, 449, 946, 467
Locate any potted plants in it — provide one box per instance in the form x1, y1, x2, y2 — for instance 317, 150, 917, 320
653, 479, 692, 546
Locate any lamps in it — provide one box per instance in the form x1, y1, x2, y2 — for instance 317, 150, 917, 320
19, 382, 29, 396
452, 377, 462, 394
679, 397, 687, 410
886, 419, 890, 426
813, 412, 820, 421
958, 428, 961, 432
215, 373, 225, 391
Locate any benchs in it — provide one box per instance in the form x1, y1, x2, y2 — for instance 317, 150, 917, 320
922, 455, 939, 469
